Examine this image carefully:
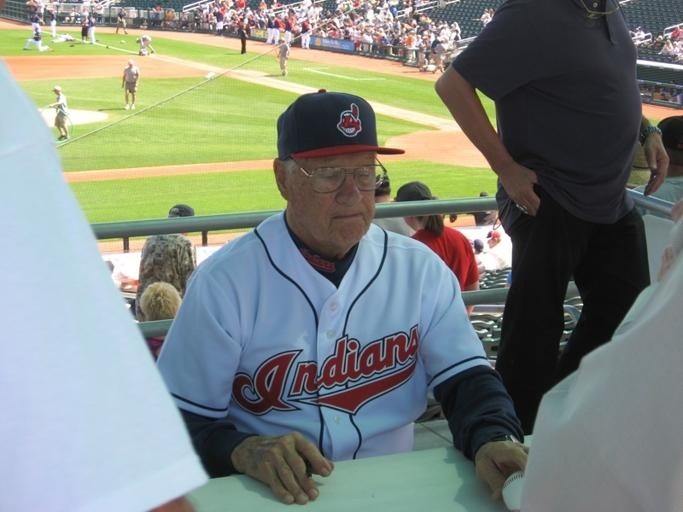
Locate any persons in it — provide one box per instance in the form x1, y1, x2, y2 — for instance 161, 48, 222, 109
629, 24, 682, 62
641, 81, 683, 105
632, 115, 683, 217
24, 1, 155, 55
373, 174, 480, 316
48, 86, 68, 140
106, 261, 122, 287
502, 217, 682, 511
120, 59, 140, 109
481, 8, 494, 27
467, 191, 512, 271
135, 204, 199, 359
157, 90, 529, 504
153, 0, 461, 76
0, 64, 209, 511
432, 1, 670, 441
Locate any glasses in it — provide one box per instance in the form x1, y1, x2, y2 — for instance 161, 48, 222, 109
579, 0, 620, 20
287, 156, 388, 193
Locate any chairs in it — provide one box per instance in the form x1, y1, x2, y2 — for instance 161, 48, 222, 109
111, 0, 339, 21
463, 263, 584, 357
410, 1, 681, 62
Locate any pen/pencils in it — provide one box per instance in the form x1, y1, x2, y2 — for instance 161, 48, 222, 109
305, 460, 312, 480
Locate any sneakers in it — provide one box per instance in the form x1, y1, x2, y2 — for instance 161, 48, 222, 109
151, 50, 156, 53
65, 136, 70, 139
131, 105, 136, 110
125, 104, 130, 110
59, 135, 66, 141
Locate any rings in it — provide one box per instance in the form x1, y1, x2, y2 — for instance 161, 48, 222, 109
515, 203, 526, 212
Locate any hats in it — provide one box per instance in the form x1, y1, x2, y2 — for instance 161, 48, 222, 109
168, 204, 195, 216
275, 89, 406, 160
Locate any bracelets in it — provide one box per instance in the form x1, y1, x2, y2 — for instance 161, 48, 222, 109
491, 434, 518, 442
638, 126, 661, 146
